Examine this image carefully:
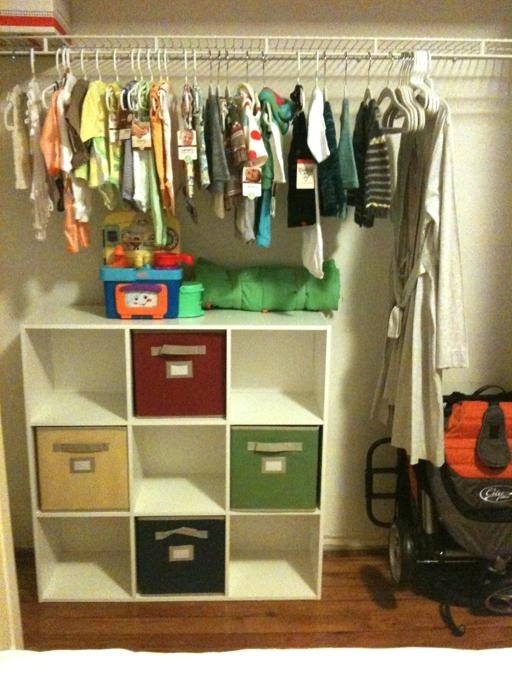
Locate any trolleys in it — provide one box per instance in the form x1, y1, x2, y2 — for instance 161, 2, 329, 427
365, 388, 511, 615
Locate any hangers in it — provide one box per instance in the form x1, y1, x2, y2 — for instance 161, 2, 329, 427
41, 49, 439, 133
26, 48, 44, 125
3, 49, 21, 131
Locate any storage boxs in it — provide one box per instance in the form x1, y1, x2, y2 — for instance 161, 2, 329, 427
230, 426, 319, 513
98, 264, 183, 321
0, 0, 72, 46
135, 515, 224, 596
131, 329, 226, 418
35, 426, 129, 513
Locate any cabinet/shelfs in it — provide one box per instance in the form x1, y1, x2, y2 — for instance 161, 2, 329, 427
18, 326, 329, 603
